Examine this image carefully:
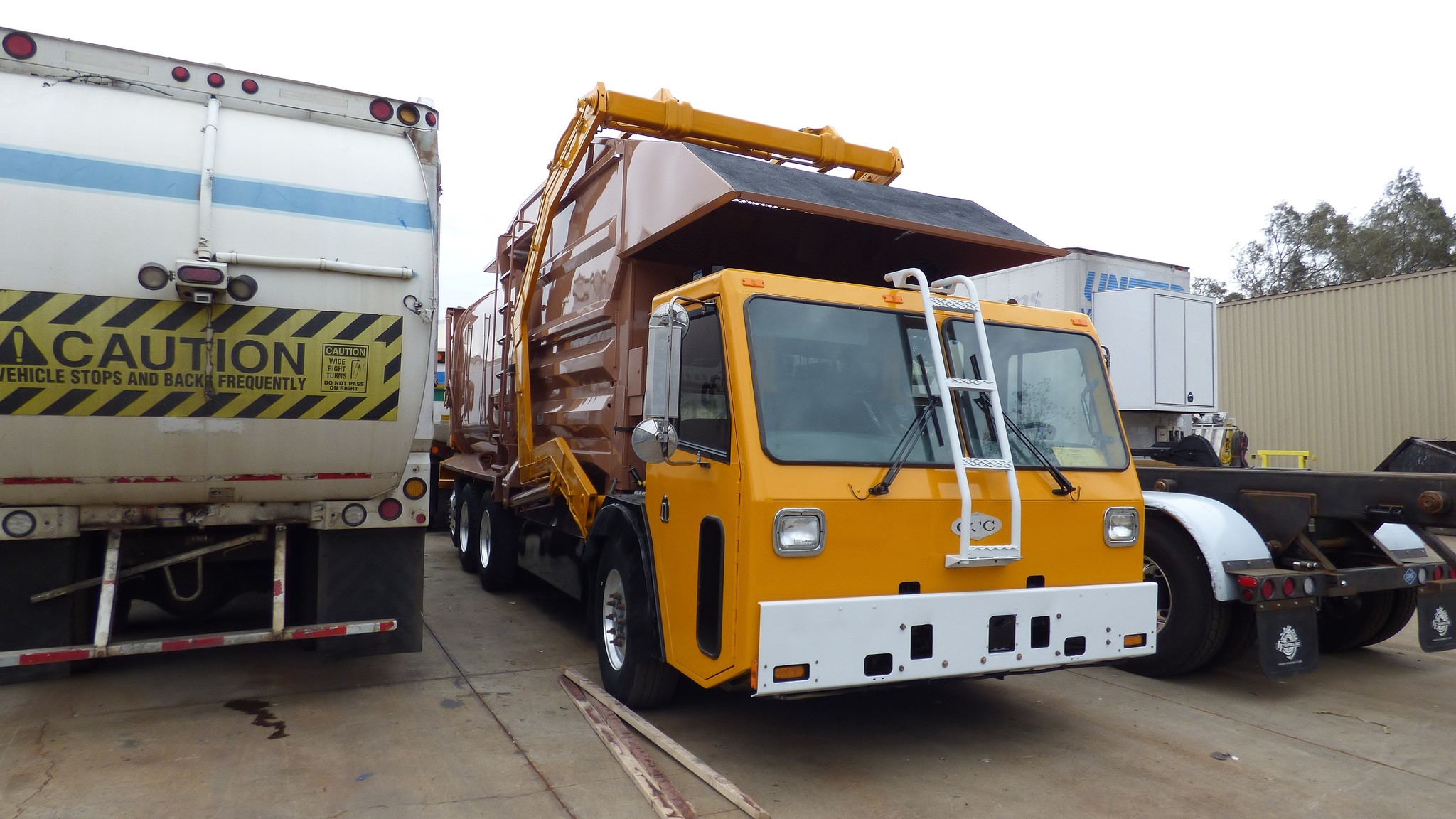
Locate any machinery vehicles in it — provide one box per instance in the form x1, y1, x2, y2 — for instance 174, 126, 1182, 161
0, 25, 446, 668
430, 82, 1156, 716
951, 251, 1456, 680
